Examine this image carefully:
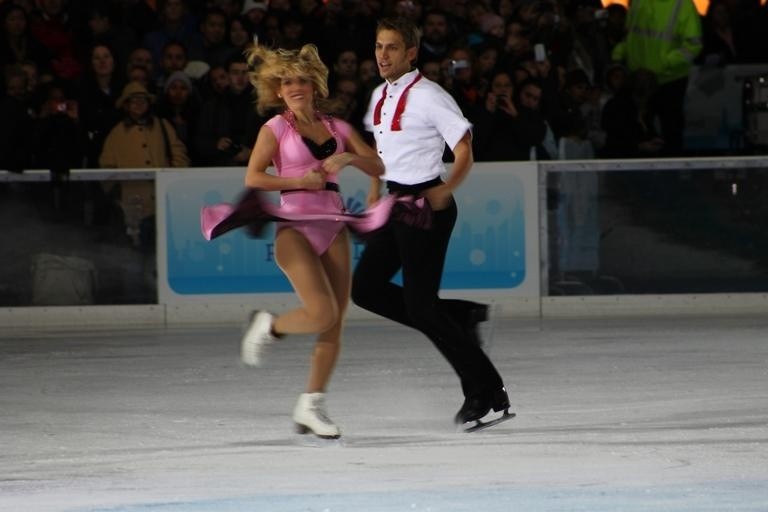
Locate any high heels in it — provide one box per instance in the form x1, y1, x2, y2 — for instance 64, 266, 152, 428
293, 392, 341, 439
240, 310, 278, 367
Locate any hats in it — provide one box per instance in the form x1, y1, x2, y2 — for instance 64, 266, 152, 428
114, 81, 157, 108
165, 71, 193, 91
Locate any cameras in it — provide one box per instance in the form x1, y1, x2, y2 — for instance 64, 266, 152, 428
447, 60, 469, 77
47, 100, 69, 113
496, 94, 506, 105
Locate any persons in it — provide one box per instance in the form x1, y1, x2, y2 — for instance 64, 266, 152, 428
350, 13, 513, 426
2, 1, 766, 291
200, 35, 386, 438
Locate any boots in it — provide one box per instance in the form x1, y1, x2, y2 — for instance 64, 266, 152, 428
452, 300, 510, 424
